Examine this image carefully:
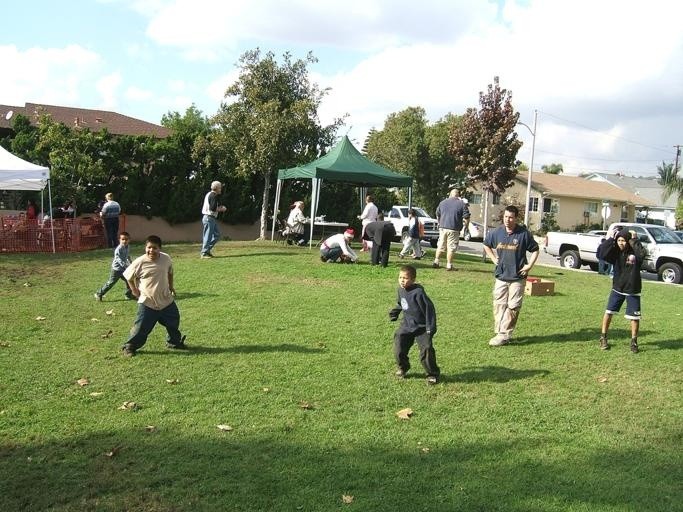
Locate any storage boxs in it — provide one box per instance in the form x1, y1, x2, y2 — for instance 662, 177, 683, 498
523, 277, 555, 296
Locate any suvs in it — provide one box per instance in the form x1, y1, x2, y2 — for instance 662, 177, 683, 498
384, 205, 439, 248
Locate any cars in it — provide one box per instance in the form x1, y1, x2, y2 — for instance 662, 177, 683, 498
459, 219, 488, 242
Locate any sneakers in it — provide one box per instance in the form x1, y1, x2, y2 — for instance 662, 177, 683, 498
446, 265, 452, 271
123, 343, 136, 357
432, 263, 439, 268
201, 255, 214, 259
489, 334, 509, 345
599, 337, 609, 350
630, 342, 638, 353
396, 363, 410, 377
166, 336, 188, 349
94, 292, 102, 302
428, 376, 438, 385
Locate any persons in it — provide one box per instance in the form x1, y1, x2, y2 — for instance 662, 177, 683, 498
605, 226, 624, 277
283, 194, 427, 267
595, 227, 648, 354
596, 238, 607, 275
120, 233, 189, 356
94, 230, 134, 303
387, 263, 441, 386
432, 188, 471, 271
197, 179, 227, 259
483, 205, 540, 346
17, 192, 120, 249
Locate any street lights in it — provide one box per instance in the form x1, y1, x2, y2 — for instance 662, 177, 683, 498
517, 119, 536, 231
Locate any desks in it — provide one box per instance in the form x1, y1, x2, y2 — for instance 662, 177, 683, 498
310, 221, 348, 248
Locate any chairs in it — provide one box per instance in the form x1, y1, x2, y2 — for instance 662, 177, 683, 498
0, 214, 102, 253
281, 218, 302, 246
274, 218, 282, 244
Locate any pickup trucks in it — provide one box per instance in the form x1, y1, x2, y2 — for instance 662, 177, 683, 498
542, 220, 683, 283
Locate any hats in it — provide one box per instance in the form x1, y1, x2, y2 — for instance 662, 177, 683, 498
344, 229, 355, 239
